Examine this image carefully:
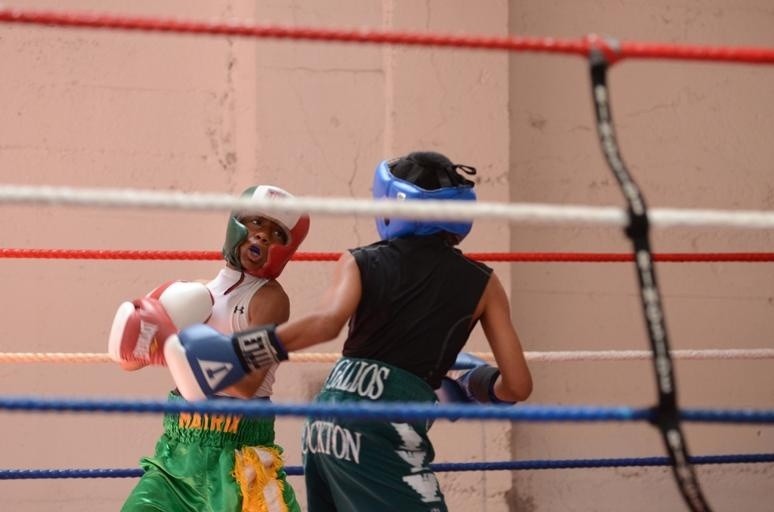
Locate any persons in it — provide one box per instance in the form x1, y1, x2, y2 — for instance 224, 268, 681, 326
108, 184, 310, 512
163, 153, 533, 510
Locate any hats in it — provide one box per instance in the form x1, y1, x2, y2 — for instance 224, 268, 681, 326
371, 154, 478, 242
221, 184, 311, 281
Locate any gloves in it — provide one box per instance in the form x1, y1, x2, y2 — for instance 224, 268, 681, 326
144, 279, 215, 333
433, 350, 517, 423
107, 296, 181, 372
163, 321, 290, 403
